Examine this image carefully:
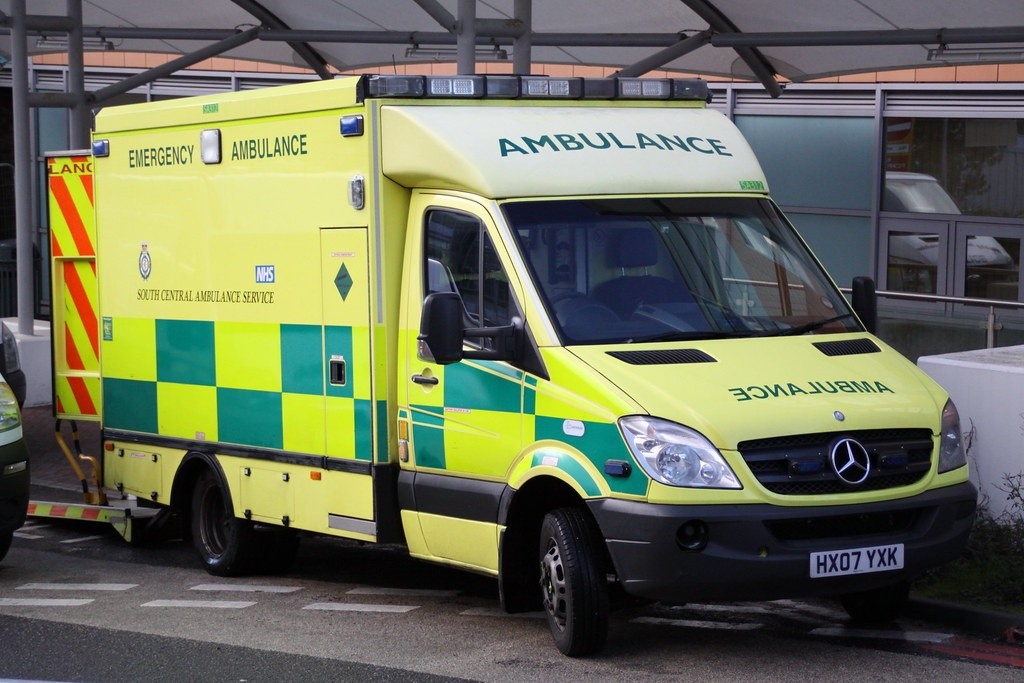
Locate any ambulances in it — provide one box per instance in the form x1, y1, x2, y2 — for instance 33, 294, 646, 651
46, 71, 978, 661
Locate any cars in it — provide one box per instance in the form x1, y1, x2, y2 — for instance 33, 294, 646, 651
0, 321, 31, 565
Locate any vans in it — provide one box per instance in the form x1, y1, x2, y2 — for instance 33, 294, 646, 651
882, 170, 1013, 301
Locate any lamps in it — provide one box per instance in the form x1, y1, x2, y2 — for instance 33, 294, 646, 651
404, 45, 508, 60
35, 35, 115, 51
927, 48, 1024, 62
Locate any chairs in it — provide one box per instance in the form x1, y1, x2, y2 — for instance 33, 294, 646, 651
449, 221, 509, 325
590, 226, 676, 319
